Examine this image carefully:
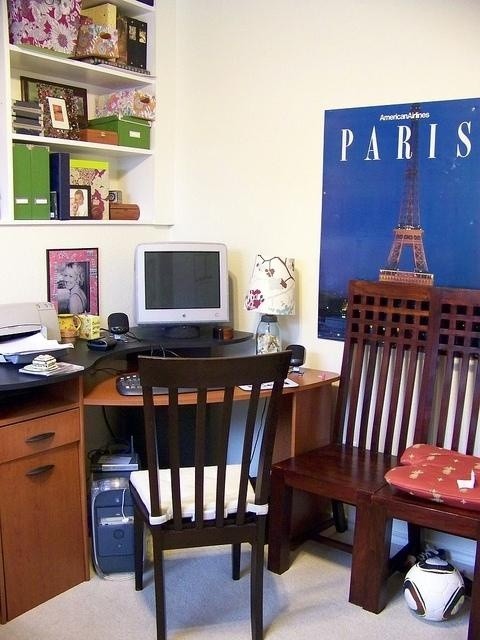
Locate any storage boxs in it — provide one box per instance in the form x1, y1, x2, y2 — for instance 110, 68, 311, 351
87, 115, 151, 150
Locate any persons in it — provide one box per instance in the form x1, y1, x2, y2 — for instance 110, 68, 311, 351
63, 263, 88, 314
70, 190, 86, 216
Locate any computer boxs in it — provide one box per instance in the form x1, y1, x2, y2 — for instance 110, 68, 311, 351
88, 470, 147, 582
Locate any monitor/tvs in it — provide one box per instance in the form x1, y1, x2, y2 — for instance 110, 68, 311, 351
134, 242, 230, 339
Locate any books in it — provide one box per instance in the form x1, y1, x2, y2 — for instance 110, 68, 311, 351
86, 4, 150, 79
12, 97, 43, 136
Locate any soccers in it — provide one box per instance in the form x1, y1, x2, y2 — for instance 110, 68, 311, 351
403, 558, 466, 621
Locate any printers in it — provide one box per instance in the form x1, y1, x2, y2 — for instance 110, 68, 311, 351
0, 301, 71, 364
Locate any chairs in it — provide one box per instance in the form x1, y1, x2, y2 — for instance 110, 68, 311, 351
362, 287, 480, 640
129, 349, 292, 640
266, 279, 435, 606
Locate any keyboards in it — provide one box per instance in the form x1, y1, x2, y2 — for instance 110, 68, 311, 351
115, 372, 226, 396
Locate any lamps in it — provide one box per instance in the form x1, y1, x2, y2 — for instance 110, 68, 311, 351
243, 248, 295, 355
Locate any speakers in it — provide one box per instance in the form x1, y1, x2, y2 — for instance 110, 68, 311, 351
286, 345, 305, 374
108, 312, 129, 341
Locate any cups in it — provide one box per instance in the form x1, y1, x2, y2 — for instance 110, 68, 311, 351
58, 314, 82, 338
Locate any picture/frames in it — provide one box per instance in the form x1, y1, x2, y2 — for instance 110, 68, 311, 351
20, 75, 88, 129
69, 185, 92, 220
37, 83, 81, 141
46, 248, 99, 317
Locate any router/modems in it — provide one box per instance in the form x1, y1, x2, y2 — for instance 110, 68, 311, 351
91, 435, 140, 471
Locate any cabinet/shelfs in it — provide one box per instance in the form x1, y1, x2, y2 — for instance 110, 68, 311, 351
0, 326, 340, 626
0, 0, 174, 226
